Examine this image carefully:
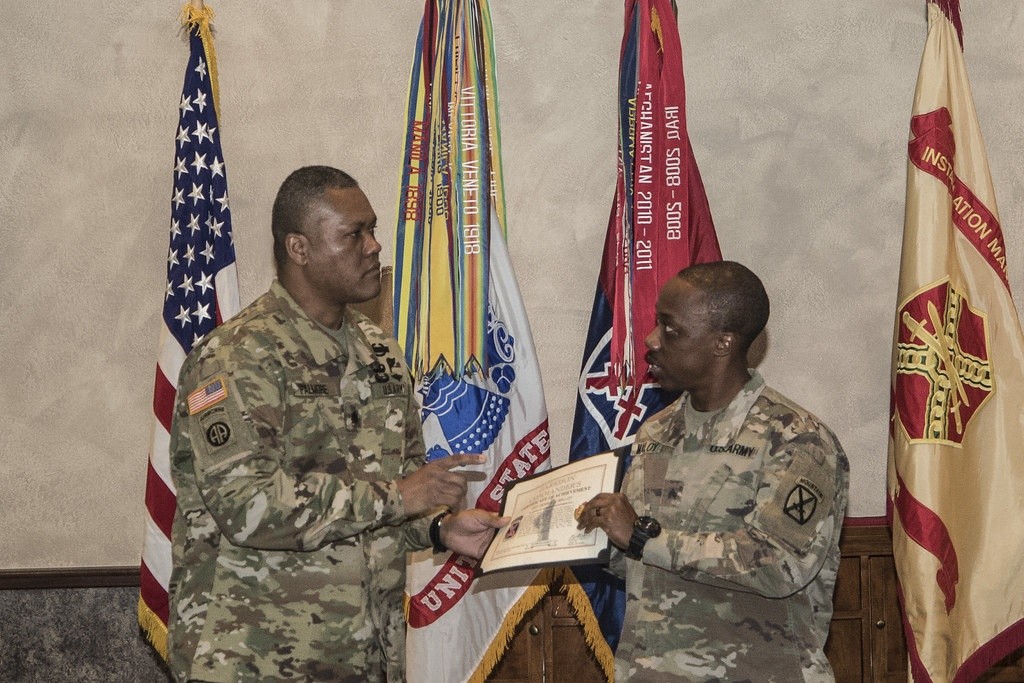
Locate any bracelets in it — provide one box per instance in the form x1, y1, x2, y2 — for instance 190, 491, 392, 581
429, 511, 452, 553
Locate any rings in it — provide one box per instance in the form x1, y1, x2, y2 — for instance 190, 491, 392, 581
596, 508, 600, 515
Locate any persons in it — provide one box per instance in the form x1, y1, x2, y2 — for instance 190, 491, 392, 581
576, 261, 849, 683
171, 166, 512, 683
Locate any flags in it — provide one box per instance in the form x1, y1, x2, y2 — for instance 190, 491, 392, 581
886, 1, 1024, 683
391, 0, 565, 683
559, 0, 723, 682
140, 4, 239, 667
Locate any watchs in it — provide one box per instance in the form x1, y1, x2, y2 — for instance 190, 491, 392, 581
625, 516, 662, 561
